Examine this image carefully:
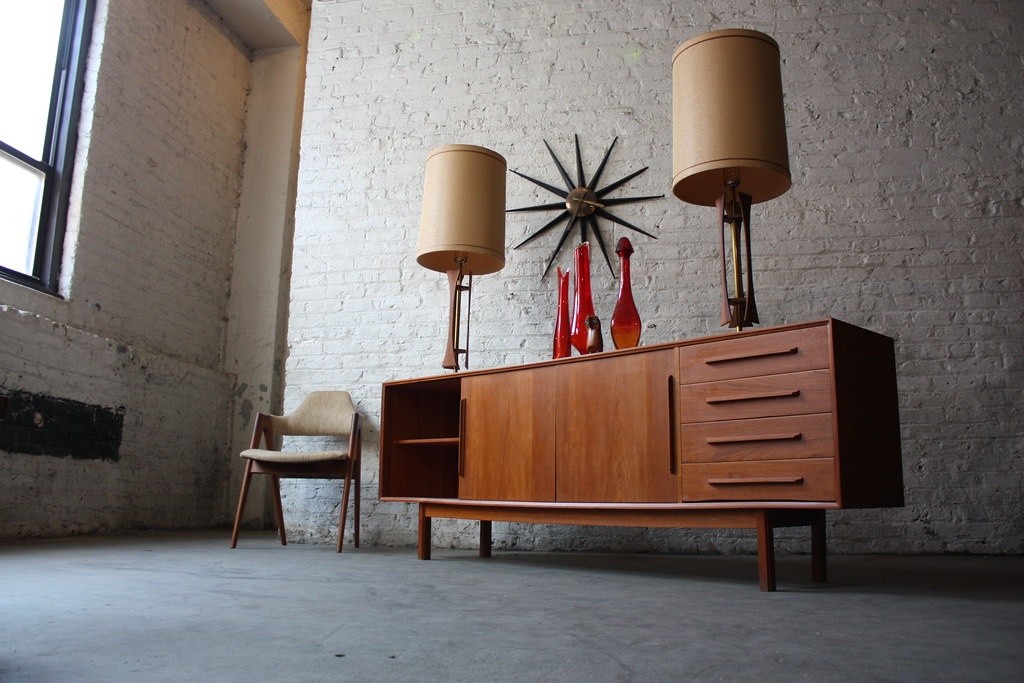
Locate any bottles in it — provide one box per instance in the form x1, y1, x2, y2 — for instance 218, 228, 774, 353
610, 237, 641, 350
553, 267, 571, 359
570, 243, 595, 355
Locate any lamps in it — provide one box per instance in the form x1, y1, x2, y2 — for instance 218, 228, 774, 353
415, 145, 507, 374
670, 28, 791, 331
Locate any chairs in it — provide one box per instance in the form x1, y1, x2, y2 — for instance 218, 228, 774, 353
229, 392, 361, 553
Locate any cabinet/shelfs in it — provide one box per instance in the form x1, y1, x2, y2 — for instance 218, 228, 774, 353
378, 359, 555, 560
680, 314, 905, 592
556, 341, 683, 528
